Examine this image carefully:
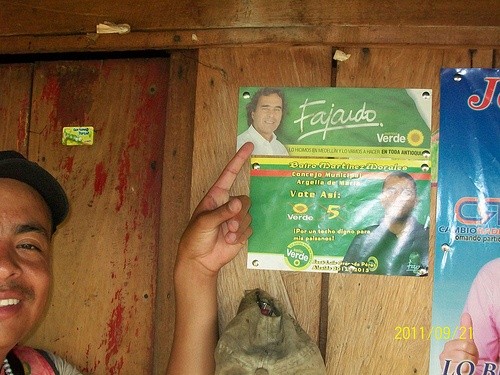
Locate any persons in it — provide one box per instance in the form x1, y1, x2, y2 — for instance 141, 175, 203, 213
339, 171, 428, 277
237, 87, 290, 156
438, 255, 500, 375
1, 141, 257, 375
